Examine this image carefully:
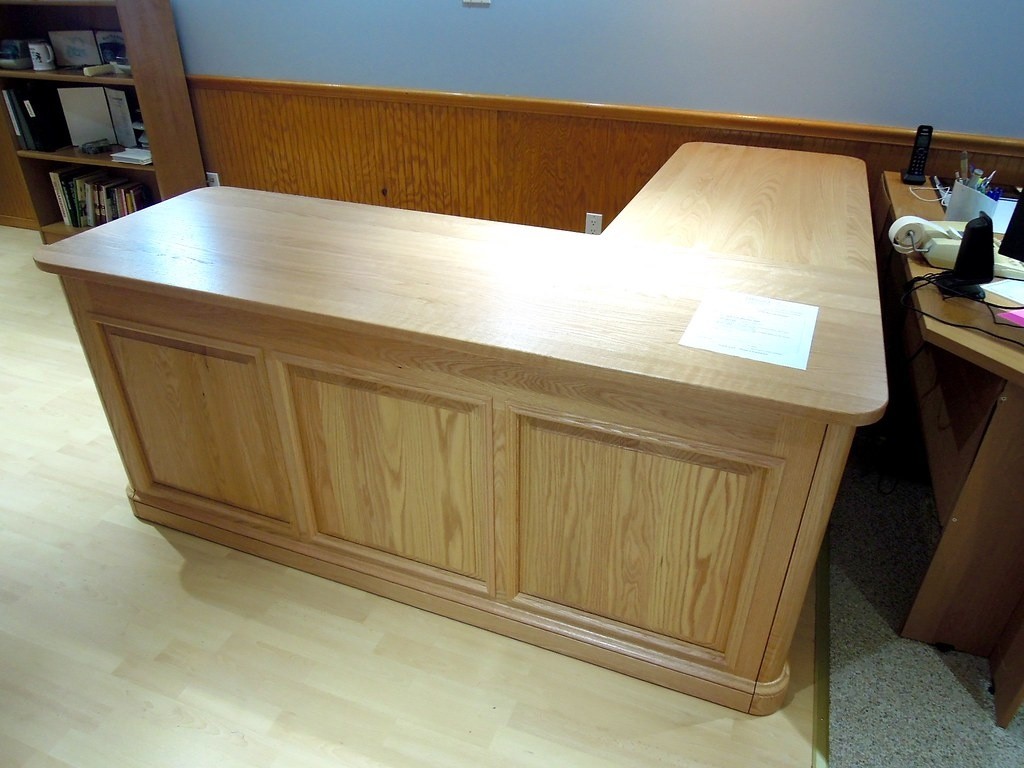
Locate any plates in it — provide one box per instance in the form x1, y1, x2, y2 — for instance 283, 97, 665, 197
0, 57, 31, 70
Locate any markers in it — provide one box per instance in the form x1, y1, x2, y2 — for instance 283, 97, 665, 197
968, 169, 983, 188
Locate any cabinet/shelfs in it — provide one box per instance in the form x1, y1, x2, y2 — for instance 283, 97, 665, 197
32, 139, 891, 720
0, 0, 208, 246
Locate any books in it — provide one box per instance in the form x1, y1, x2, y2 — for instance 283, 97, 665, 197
2, 82, 137, 153
49, 164, 153, 228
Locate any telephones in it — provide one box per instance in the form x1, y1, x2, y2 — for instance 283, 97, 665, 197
900, 126, 933, 185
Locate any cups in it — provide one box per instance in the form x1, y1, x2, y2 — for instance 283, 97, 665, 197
943, 177, 997, 229
28, 39, 56, 71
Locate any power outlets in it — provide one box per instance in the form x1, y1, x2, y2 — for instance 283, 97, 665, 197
206, 172, 220, 187
585, 213, 602, 235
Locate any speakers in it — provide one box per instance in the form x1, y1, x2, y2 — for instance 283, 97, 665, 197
934, 210, 994, 301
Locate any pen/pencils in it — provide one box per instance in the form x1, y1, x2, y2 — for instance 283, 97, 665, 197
979, 170, 996, 190
986, 184, 1002, 201
956, 171, 969, 185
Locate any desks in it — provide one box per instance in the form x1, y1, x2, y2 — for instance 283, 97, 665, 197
870, 170, 1024, 730
